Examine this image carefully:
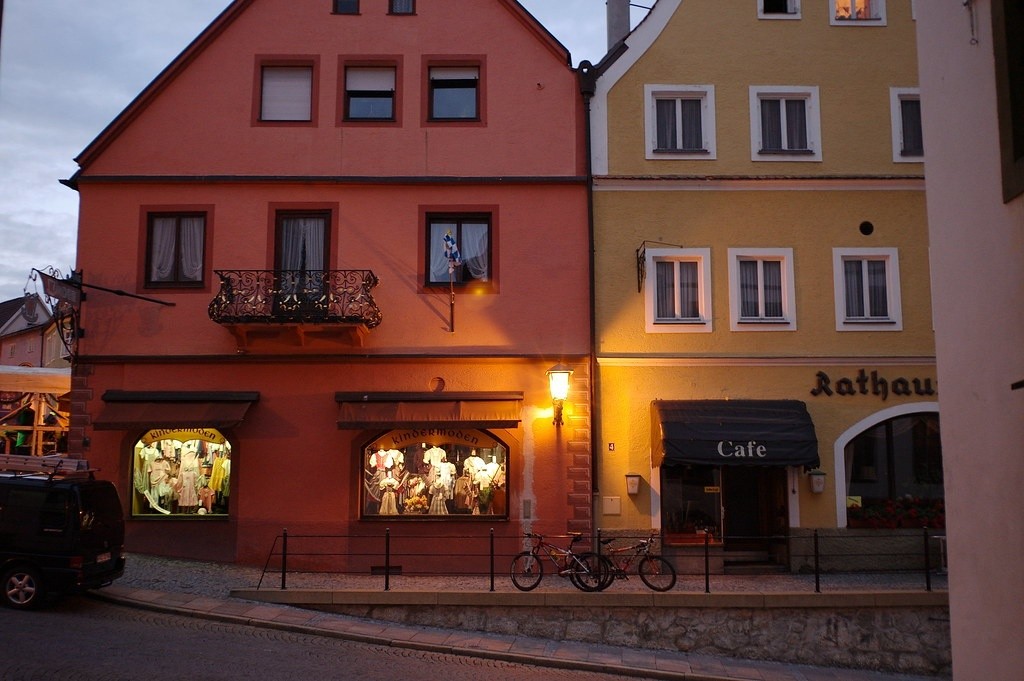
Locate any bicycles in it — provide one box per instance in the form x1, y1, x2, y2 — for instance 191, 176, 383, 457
579, 528, 677, 591
509, 530, 608, 592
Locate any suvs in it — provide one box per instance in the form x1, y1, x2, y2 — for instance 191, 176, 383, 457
0, 447, 128, 609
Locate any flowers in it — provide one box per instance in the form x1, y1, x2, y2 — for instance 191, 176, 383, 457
846, 494, 945, 528
405, 494, 429, 513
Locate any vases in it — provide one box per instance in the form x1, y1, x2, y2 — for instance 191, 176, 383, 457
410, 510, 423, 515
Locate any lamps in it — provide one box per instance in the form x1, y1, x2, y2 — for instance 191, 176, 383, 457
808, 470, 826, 495
625, 471, 641, 496
545, 358, 575, 428
